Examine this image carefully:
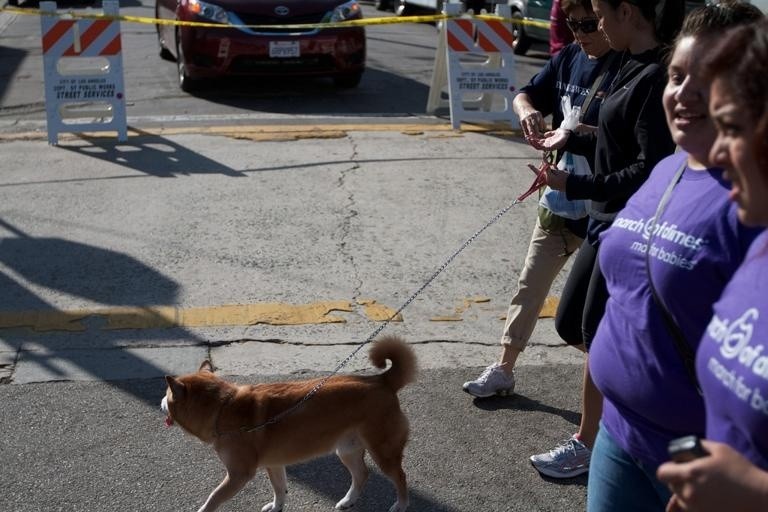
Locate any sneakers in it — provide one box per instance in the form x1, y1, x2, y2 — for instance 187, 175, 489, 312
529, 433, 591, 479
462, 363, 515, 398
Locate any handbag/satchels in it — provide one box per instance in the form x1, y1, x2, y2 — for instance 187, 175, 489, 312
538, 147, 560, 230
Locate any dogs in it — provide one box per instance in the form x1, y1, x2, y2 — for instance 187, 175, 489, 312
159, 333, 419, 512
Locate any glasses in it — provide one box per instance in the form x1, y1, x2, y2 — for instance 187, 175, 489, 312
564, 16, 598, 33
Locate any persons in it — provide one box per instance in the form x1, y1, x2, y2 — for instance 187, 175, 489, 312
461, 0, 704, 478
656, 22, 765, 511
587, 0, 767, 512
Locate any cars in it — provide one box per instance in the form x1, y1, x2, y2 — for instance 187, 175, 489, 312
152, 1, 368, 101
375, 1, 497, 17
506, 1, 552, 55
